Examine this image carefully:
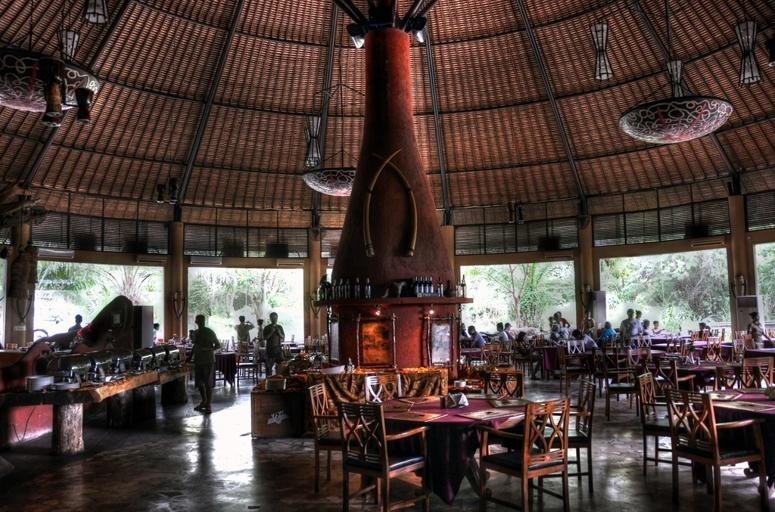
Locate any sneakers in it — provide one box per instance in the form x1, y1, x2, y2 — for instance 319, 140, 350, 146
194, 406, 211, 413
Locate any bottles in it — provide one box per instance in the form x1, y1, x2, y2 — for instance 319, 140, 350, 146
456, 277, 462, 296
218, 339, 230, 352
444, 280, 455, 297
346, 357, 352, 373
272, 363, 278, 376
283, 343, 291, 357
305, 334, 328, 355
461, 273, 467, 297
439, 396, 446, 408
413, 275, 443, 298
317, 276, 371, 299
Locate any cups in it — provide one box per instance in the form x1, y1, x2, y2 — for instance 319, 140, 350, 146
454, 381, 465, 388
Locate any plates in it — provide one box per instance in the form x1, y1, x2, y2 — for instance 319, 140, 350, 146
53, 382, 81, 391
24, 374, 55, 392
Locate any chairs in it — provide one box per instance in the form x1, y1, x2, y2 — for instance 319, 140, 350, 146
528, 378, 598, 502
456, 329, 774, 419
478, 397, 572, 510
251, 363, 449, 441
307, 383, 370, 498
635, 372, 774, 511
183, 335, 330, 387
335, 398, 429, 511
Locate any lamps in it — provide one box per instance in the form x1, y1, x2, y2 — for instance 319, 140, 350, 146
0, 0, 116, 115
295, 62, 374, 201
584, 0, 768, 147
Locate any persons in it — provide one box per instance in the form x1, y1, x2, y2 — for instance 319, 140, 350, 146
698, 322, 712, 338
253, 318, 266, 347
68, 314, 83, 332
548, 310, 617, 350
153, 323, 159, 339
619, 309, 665, 347
263, 311, 285, 376
461, 322, 514, 349
234, 315, 255, 352
185, 315, 221, 414
518, 331, 543, 378
746, 311, 772, 349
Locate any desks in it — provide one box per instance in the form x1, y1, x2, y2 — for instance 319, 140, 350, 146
378, 395, 537, 506
